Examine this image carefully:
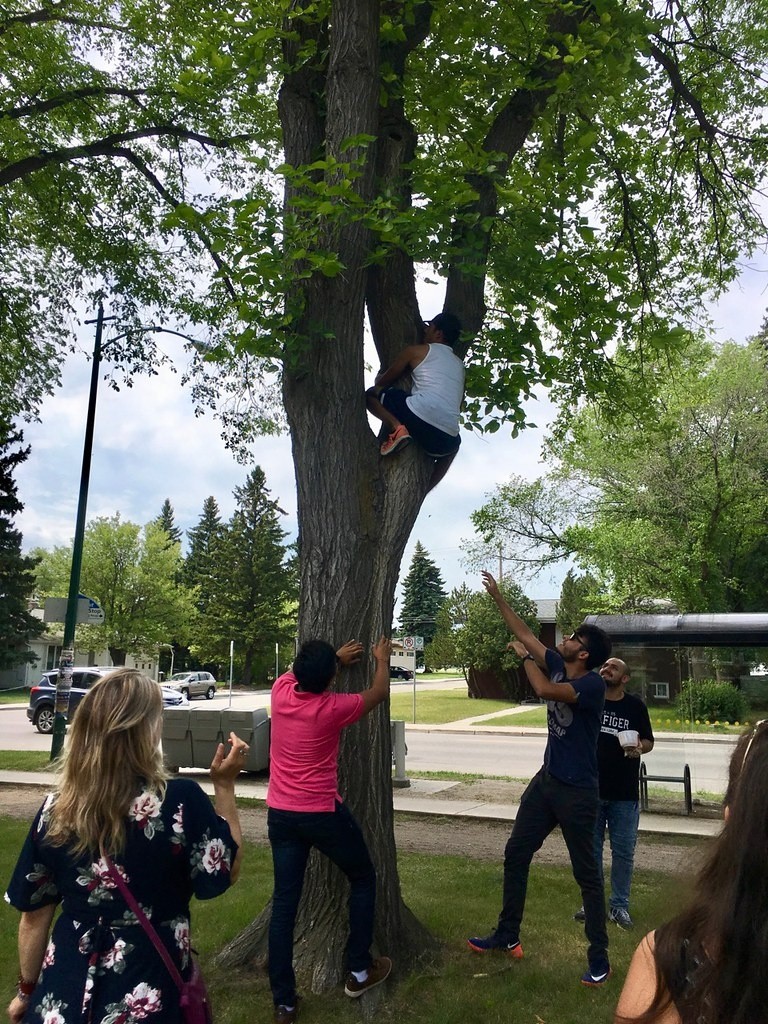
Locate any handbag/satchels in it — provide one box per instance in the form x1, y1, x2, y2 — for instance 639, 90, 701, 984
179, 961, 213, 1024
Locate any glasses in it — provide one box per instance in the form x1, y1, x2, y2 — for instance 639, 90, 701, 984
739, 718, 768, 774
569, 632, 589, 652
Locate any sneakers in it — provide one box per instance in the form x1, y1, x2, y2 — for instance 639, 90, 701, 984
467, 928, 524, 958
607, 905, 634, 932
345, 957, 392, 997
580, 967, 613, 987
572, 906, 585, 923
274, 995, 302, 1024
380, 425, 412, 456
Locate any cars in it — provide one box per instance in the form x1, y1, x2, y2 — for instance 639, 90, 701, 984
389, 666, 414, 681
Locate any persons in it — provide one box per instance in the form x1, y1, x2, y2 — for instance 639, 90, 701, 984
467, 569, 611, 987
366, 312, 468, 492
614, 721, 768, 1024
3, 669, 250, 1024
575, 657, 653, 931
264, 636, 394, 1024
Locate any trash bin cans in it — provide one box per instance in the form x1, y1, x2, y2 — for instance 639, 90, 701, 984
162, 705, 270, 772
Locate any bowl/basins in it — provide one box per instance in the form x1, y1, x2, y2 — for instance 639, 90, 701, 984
616, 730, 639, 751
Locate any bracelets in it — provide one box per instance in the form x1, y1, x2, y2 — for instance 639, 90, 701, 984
18, 970, 37, 1002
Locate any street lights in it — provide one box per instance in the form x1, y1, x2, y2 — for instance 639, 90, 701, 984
48, 298, 212, 762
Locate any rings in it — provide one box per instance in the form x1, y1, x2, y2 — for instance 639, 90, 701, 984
240, 749, 245, 755
244, 752, 249, 757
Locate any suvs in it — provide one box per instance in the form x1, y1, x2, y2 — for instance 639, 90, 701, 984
26, 666, 190, 734
159, 670, 217, 701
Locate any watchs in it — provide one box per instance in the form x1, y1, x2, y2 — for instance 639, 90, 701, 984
522, 654, 535, 663
